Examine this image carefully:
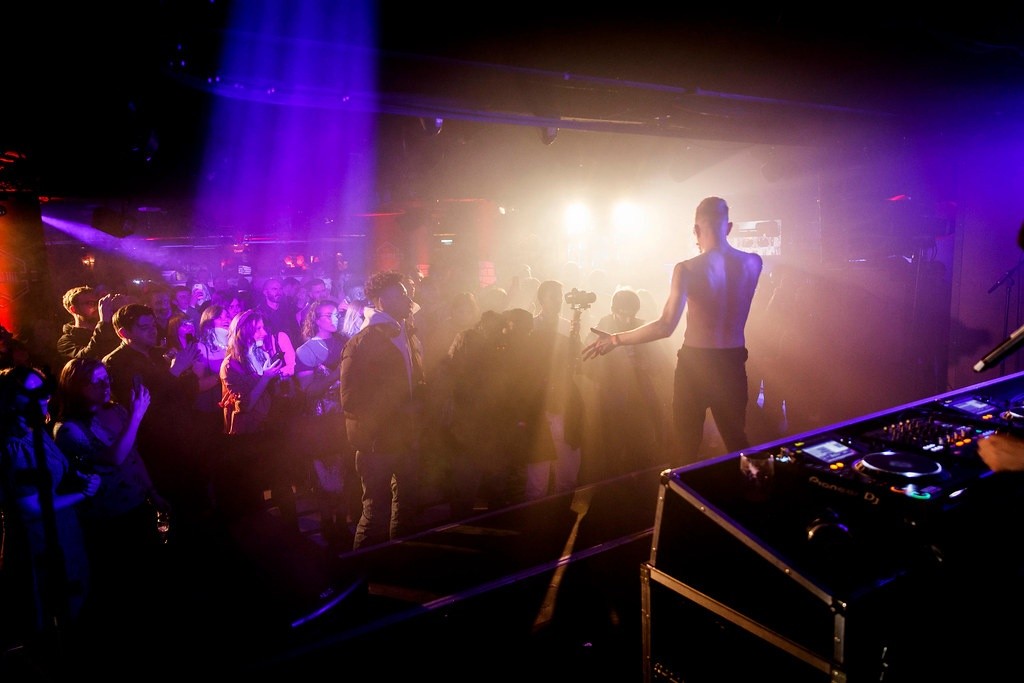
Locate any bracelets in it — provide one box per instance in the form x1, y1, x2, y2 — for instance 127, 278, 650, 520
613, 333, 623, 346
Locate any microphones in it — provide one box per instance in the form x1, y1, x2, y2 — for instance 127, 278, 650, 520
973, 323, 1024, 373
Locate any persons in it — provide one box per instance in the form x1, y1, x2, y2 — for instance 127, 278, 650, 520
578, 196, 762, 464
976, 434, 1024, 473
0, 247, 654, 648
339, 270, 420, 551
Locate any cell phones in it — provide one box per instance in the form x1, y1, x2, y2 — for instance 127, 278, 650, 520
195, 284, 203, 299
132, 375, 142, 399
271, 352, 285, 365
185, 334, 192, 345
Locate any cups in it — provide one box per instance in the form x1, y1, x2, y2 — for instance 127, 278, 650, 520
740, 451, 775, 506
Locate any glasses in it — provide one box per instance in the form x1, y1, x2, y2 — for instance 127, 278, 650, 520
79, 301, 99, 308
317, 313, 342, 321
